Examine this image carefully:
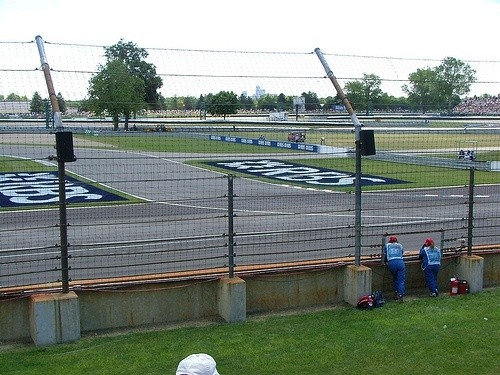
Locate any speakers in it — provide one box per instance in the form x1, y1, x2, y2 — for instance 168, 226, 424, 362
56, 132, 74, 162
359, 130, 375, 156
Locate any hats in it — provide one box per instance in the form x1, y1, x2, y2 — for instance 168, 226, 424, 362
425, 238, 433, 244
389, 236, 398, 242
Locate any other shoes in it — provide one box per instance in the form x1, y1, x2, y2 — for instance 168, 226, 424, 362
393, 289, 403, 302
431, 289, 439, 297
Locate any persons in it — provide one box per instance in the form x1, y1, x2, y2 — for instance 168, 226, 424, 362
140, 109, 407, 117
381, 236, 405, 304
259, 135, 266, 141
176, 353, 219, 375
458, 150, 475, 161
419, 238, 441, 297
155, 123, 166, 132
290, 133, 304, 142
453, 98, 500, 115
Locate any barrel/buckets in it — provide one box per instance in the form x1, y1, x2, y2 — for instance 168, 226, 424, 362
459, 279, 469, 295
450, 277, 459, 296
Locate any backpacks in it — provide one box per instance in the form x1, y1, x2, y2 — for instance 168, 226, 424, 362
357, 290, 385, 310
